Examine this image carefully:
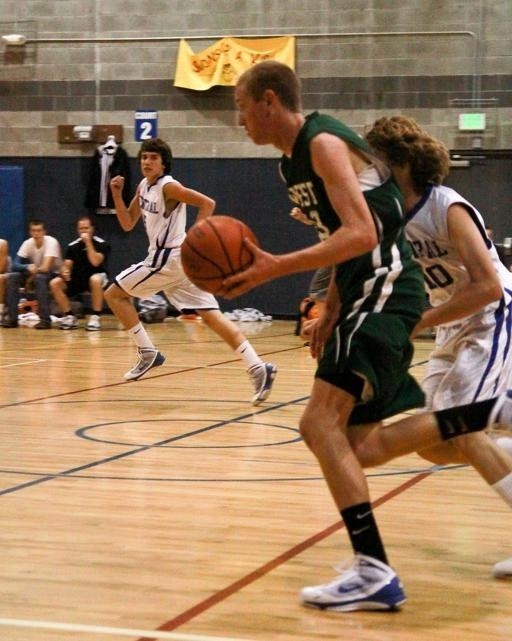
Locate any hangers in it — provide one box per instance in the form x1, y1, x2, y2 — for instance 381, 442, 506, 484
102, 135, 119, 156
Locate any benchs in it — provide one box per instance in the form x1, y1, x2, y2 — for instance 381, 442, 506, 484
16, 286, 135, 331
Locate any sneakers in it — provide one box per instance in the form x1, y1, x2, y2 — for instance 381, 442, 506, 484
59, 314, 79, 330
34, 317, 51, 329
2, 318, 18, 328
84, 314, 102, 332
300, 562, 407, 615
124, 345, 165, 382
247, 361, 276, 408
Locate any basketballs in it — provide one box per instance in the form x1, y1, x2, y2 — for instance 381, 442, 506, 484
180, 215, 260, 293
